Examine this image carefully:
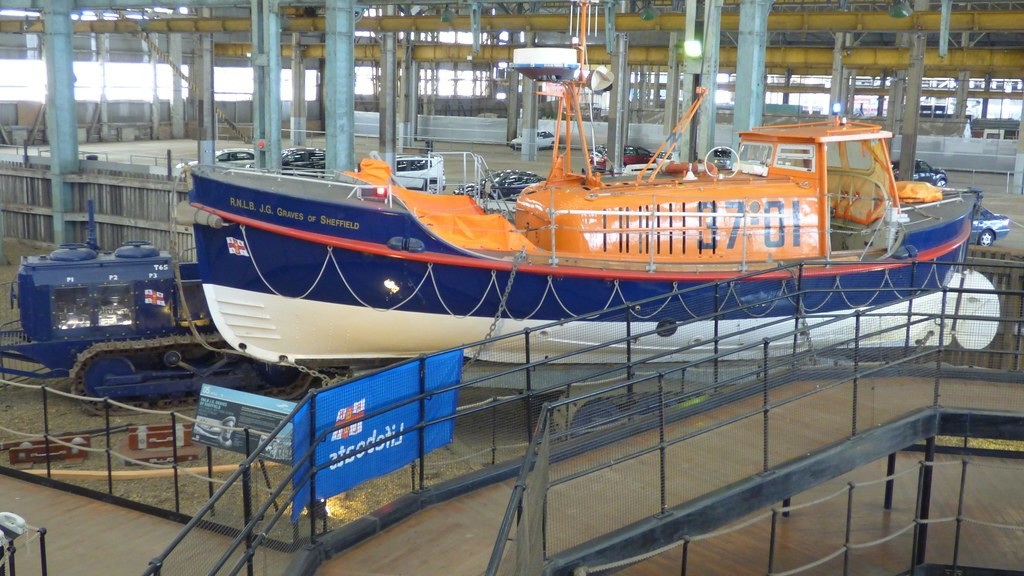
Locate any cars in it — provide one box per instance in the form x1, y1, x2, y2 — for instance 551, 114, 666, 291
174, 148, 255, 172
712, 145, 732, 172
967, 203, 1012, 247
453, 168, 547, 203
509, 128, 556, 152
280, 146, 326, 178
588, 142, 658, 176
890, 158, 948, 189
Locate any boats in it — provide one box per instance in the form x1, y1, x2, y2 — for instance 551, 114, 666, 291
172, 0, 984, 374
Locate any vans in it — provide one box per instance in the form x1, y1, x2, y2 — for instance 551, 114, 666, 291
393, 155, 446, 194
